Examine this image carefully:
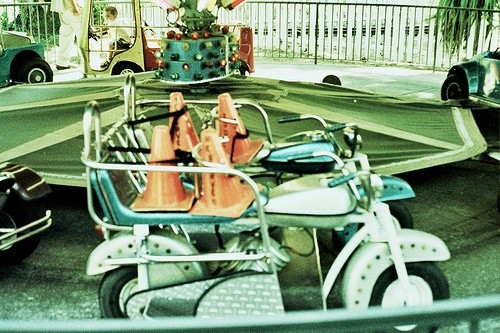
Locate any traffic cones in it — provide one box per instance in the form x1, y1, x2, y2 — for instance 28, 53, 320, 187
190, 130, 263, 219
213, 93, 266, 165
165, 92, 206, 161
130, 125, 196, 217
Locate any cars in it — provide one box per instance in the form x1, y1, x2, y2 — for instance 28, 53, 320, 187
439, 45, 500, 110
0, 27, 53, 87
0, 159, 56, 268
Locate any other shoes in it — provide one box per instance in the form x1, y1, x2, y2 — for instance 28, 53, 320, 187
100, 61, 111, 68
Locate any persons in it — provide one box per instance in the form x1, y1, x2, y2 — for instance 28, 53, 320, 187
51, 0, 91, 70
90, 5, 132, 67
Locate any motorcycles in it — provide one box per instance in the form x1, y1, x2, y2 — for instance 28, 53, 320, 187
84, 73, 452, 333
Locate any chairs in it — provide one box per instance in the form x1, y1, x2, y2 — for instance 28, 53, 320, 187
81, 72, 271, 232
